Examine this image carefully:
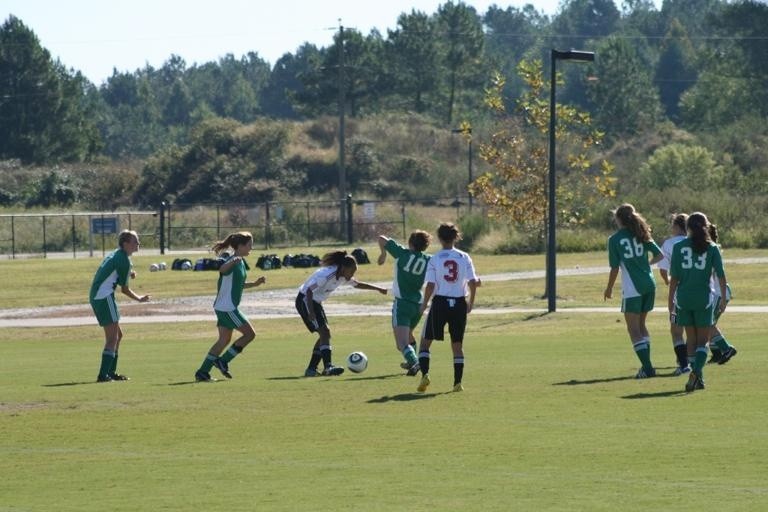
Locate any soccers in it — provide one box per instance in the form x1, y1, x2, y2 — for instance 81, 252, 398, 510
182, 262, 191, 270
347, 350, 368, 373
158, 263, 166, 270
150, 264, 158, 272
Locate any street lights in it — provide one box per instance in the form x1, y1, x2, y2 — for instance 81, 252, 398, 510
546, 47, 595, 314
450, 128, 472, 222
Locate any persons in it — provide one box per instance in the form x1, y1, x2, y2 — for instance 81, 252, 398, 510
657, 214, 689, 375
194, 231, 266, 382
377, 230, 432, 375
295, 249, 388, 377
417, 222, 477, 393
89, 229, 151, 383
668, 212, 727, 391
604, 204, 664, 378
709, 224, 736, 365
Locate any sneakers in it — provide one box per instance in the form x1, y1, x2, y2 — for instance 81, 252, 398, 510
636, 368, 655, 377
453, 383, 464, 392
108, 373, 130, 380
97, 374, 115, 382
708, 356, 723, 362
406, 357, 421, 376
720, 348, 736, 363
418, 374, 431, 391
674, 367, 689, 374
323, 364, 344, 376
686, 372, 698, 392
196, 370, 219, 382
306, 367, 323, 377
400, 363, 410, 369
213, 358, 233, 378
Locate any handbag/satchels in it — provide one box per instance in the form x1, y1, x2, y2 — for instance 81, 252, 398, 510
172, 259, 193, 270
195, 257, 228, 270
256, 254, 281, 270
352, 249, 370, 264
283, 254, 321, 267
